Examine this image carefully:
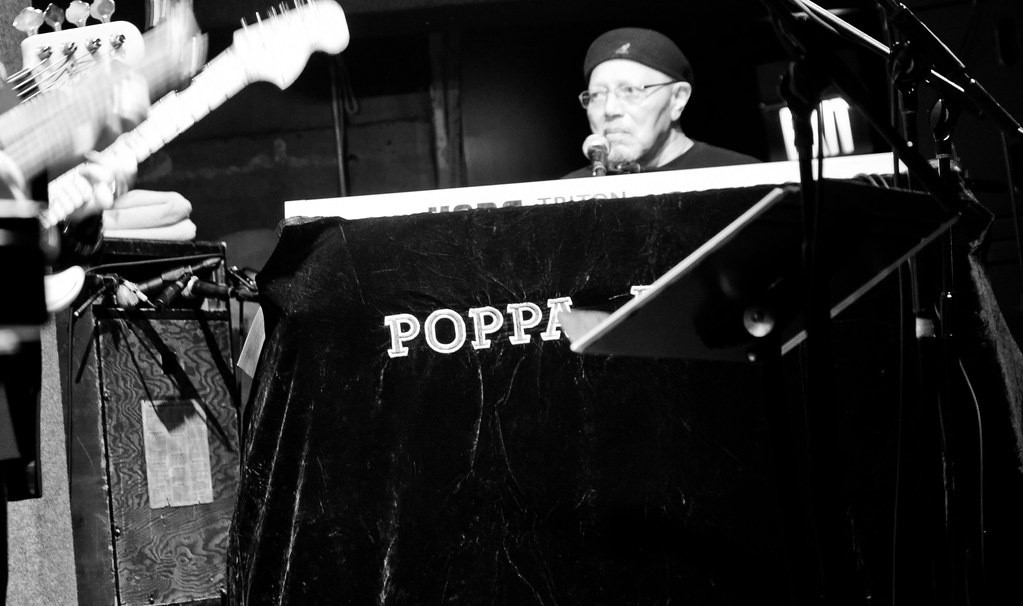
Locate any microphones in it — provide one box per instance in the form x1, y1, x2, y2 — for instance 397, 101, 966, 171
114, 258, 220, 308
582, 133, 610, 176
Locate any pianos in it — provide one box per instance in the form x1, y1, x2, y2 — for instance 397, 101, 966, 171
256, 146, 988, 606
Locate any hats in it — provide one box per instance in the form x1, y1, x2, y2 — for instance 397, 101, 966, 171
584, 28, 691, 82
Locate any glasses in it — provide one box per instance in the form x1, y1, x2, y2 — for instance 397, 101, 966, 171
578, 78, 678, 110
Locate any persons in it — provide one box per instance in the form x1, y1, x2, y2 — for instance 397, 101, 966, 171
559, 27, 767, 179
0, 64, 139, 606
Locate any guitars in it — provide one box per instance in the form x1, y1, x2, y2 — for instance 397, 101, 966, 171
6, 0, 146, 103
40, 1, 352, 232
